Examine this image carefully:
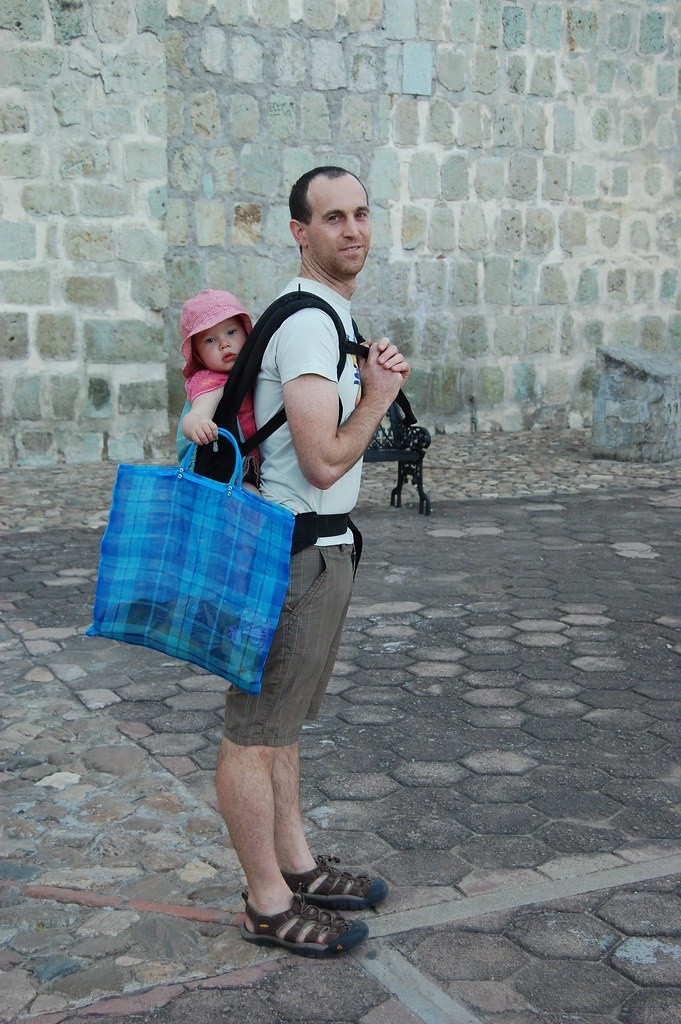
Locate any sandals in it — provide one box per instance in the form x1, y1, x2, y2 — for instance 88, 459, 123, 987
277, 856, 389, 911
241, 889, 369, 957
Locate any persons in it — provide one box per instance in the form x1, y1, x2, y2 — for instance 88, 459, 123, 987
215, 167, 408, 959
181, 290, 263, 502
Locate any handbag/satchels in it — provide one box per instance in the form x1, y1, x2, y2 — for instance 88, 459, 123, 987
84, 432, 296, 694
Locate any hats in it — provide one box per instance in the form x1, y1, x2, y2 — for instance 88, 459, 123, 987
181, 291, 254, 379
